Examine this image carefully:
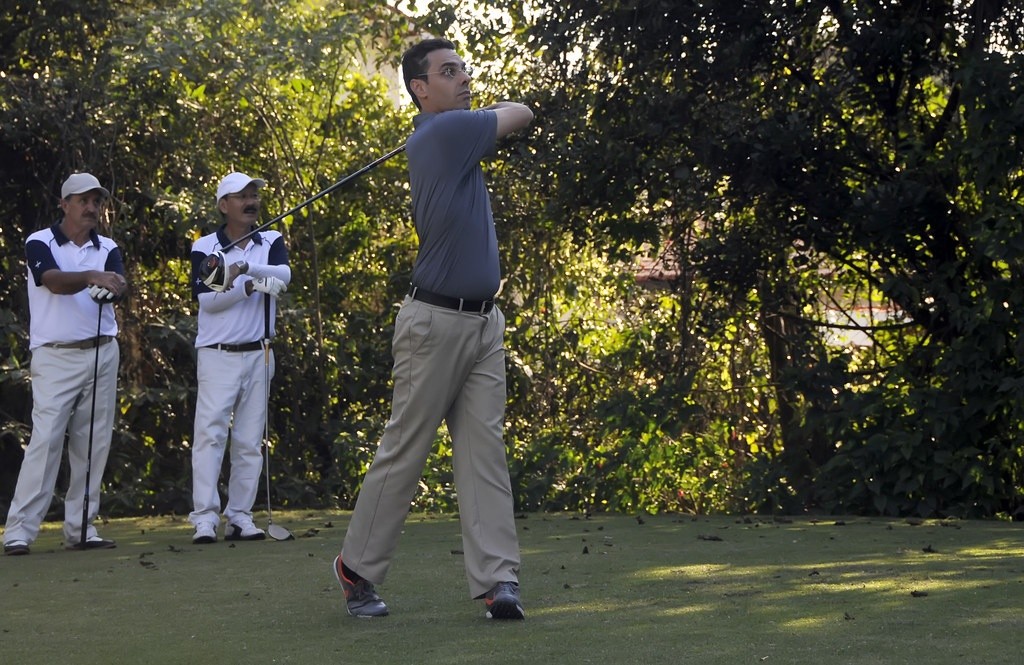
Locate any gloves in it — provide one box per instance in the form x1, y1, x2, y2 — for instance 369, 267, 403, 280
88, 275, 127, 298
245, 275, 287, 300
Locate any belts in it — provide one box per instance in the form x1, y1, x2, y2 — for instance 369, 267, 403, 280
205, 339, 266, 351
43, 335, 112, 348
409, 284, 494, 314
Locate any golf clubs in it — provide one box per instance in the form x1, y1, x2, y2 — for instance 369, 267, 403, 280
263, 293, 295, 541
78, 297, 116, 550
199, 143, 406, 293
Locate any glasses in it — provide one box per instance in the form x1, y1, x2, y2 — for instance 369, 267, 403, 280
418, 65, 470, 80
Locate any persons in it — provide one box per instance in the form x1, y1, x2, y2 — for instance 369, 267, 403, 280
334, 37, 534, 620
187, 172, 291, 544
3, 172, 127, 556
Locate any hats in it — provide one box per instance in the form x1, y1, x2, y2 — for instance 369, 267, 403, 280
217, 172, 265, 205
62, 173, 110, 203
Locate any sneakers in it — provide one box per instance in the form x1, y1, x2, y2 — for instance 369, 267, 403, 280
192, 520, 218, 545
485, 582, 526, 622
224, 515, 267, 542
74, 535, 116, 551
334, 552, 390, 620
5, 539, 31, 557
